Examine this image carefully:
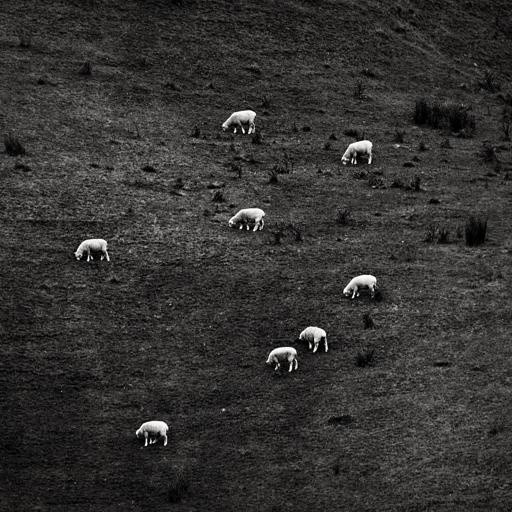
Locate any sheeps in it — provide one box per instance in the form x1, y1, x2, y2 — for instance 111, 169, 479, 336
135, 420, 172, 447
265, 346, 301, 372
220, 109, 259, 137
296, 326, 330, 355
73, 236, 111, 264
341, 272, 380, 301
226, 206, 267, 231
340, 138, 374, 167
